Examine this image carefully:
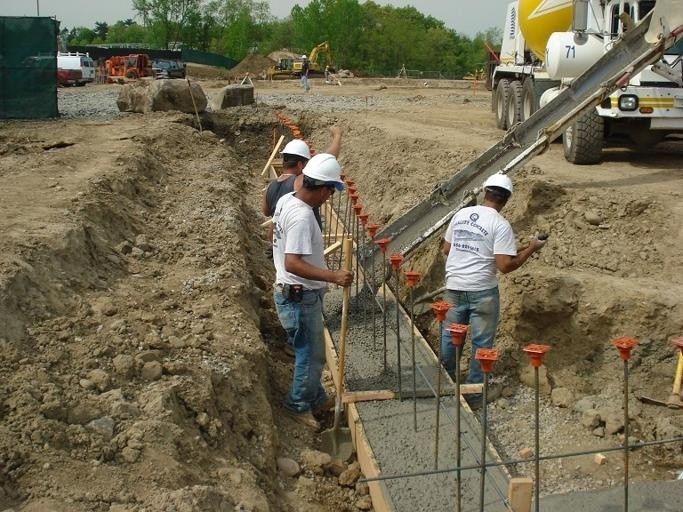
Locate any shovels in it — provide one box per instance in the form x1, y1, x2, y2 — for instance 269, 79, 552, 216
404, 233, 549, 315
321, 239, 352, 464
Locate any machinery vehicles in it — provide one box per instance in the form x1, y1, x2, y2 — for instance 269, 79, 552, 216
490, 0, 681, 166
267, 41, 333, 78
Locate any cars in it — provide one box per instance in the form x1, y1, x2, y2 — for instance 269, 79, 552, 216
152, 59, 185, 78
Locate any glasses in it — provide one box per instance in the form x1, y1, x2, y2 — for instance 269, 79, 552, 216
329, 186, 335, 196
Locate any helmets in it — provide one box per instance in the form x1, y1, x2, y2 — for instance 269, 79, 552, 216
482, 173, 513, 195
301, 153, 345, 184
279, 139, 312, 159
302, 55, 307, 58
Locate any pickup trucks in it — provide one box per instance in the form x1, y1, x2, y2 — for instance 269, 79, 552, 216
56, 68, 82, 87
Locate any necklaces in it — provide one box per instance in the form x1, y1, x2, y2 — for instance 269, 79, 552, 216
280, 173, 293, 175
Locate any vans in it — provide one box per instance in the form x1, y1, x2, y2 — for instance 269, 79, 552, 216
56, 50, 95, 88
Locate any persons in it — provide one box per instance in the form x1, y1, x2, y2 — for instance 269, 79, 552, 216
436, 174, 547, 409
300, 55, 310, 93
261, 124, 344, 356
324, 65, 331, 83
272, 154, 354, 430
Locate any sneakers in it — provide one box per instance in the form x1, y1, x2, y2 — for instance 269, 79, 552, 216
314, 393, 335, 419
280, 408, 320, 431
274, 326, 296, 355
468, 382, 503, 410
